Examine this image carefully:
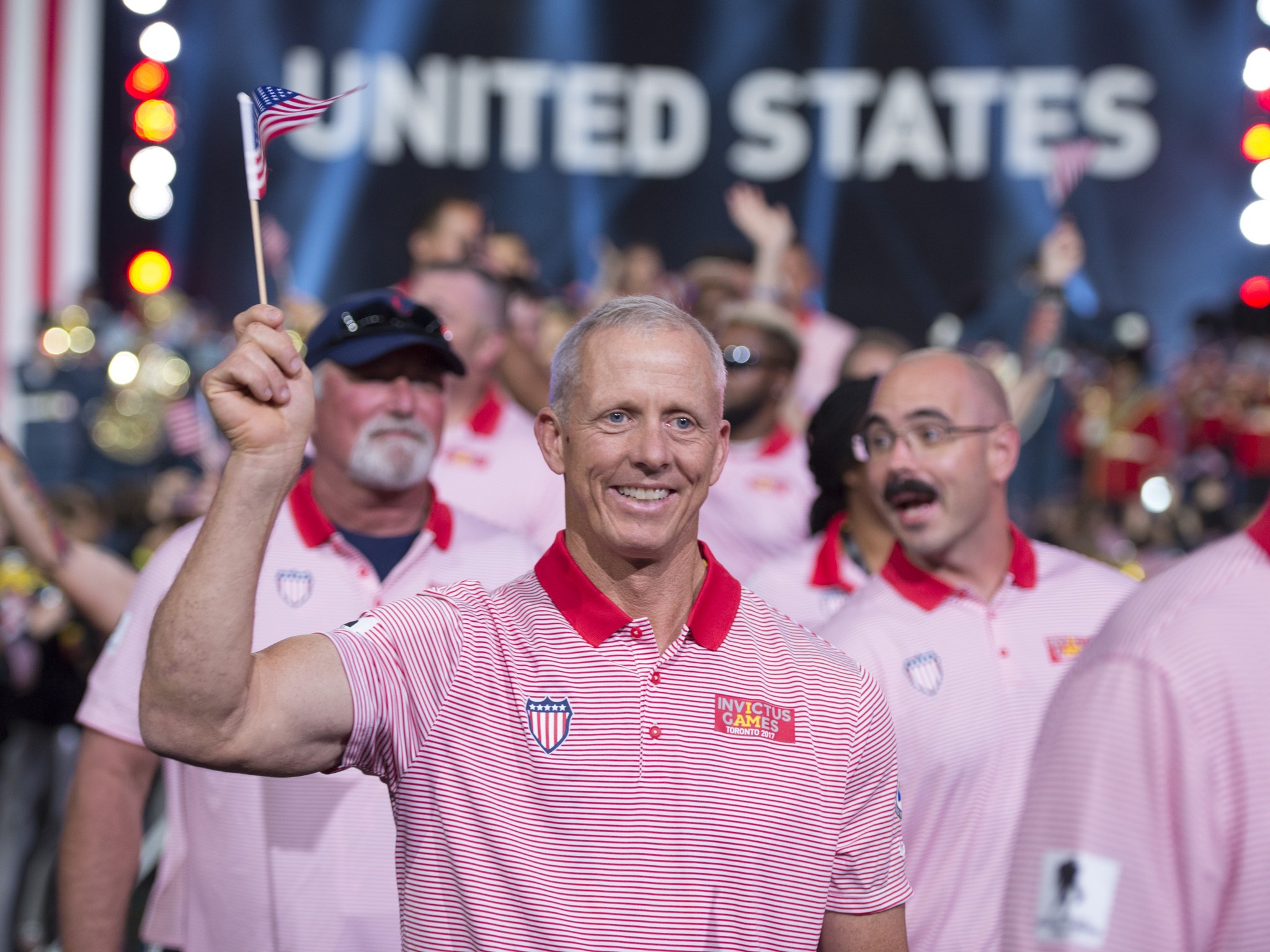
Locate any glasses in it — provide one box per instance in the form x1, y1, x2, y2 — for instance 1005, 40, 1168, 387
850, 424, 995, 459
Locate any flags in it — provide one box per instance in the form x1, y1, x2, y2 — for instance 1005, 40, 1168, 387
234, 81, 373, 200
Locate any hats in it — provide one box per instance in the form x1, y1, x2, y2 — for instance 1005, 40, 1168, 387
298, 287, 470, 377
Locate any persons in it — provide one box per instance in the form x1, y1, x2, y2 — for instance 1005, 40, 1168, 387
1002, 504, 1269, 952
58, 287, 543, 952
0, 306, 138, 950
817, 346, 1144, 952
138, 292, 911, 952
0, 181, 1269, 588
746, 372, 897, 630
